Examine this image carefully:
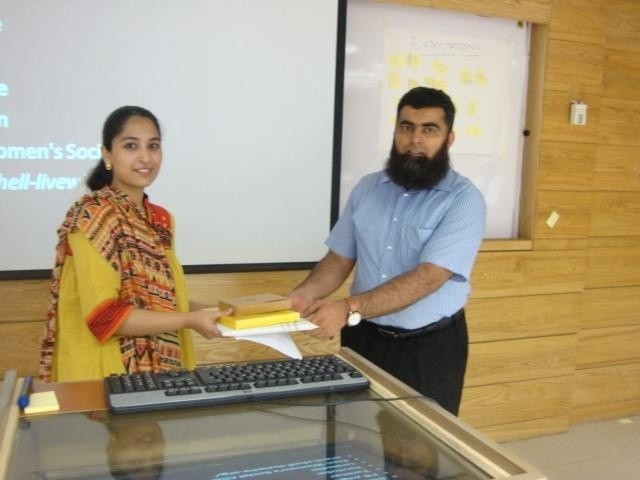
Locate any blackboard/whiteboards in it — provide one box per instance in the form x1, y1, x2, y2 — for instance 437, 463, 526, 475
340, 1, 531, 240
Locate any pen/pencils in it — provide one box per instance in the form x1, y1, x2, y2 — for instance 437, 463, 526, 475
18, 374, 33, 407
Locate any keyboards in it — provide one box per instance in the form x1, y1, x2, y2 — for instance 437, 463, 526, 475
105, 354, 370, 415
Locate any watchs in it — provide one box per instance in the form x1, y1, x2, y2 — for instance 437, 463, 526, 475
344, 296, 362, 327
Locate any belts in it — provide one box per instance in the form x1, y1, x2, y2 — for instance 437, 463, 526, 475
362, 309, 464, 341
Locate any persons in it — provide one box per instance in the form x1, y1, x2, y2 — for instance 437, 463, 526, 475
280, 87, 485, 418
39, 106, 234, 383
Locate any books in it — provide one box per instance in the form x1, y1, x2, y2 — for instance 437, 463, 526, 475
199, 293, 300, 330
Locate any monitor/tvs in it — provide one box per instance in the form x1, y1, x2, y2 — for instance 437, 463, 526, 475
62, 440, 433, 480
